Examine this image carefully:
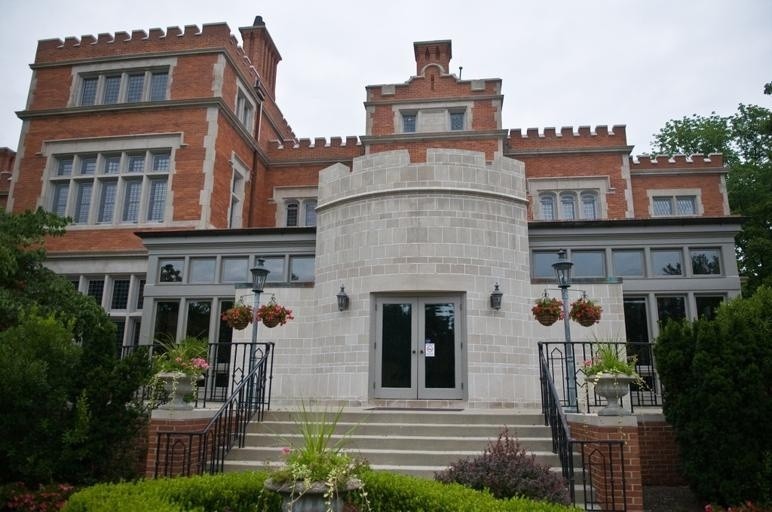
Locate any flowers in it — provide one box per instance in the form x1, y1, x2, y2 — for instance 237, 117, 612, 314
176, 355, 209, 370
531, 295, 603, 321
220, 304, 295, 326
280, 447, 293, 457
584, 359, 592, 368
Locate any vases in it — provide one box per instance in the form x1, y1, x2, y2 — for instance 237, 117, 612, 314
536, 315, 596, 327
234, 317, 280, 330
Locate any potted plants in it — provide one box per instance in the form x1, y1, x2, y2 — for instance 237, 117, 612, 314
146, 328, 211, 420
584, 332, 656, 415
256, 392, 374, 512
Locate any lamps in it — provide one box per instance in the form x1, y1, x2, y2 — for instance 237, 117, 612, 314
490, 282, 503, 311
336, 286, 349, 311
